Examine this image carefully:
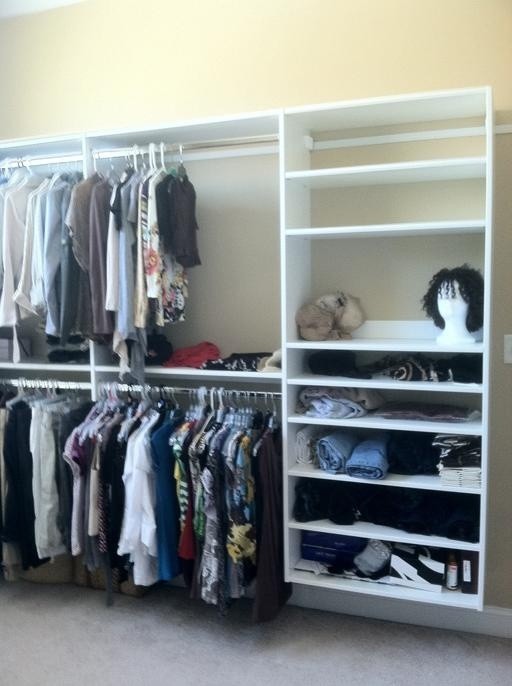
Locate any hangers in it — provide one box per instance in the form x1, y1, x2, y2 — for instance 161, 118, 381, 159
3, 141, 189, 186
1, 372, 279, 433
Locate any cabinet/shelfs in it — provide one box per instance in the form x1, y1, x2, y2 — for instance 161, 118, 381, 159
1, 85, 492, 617
